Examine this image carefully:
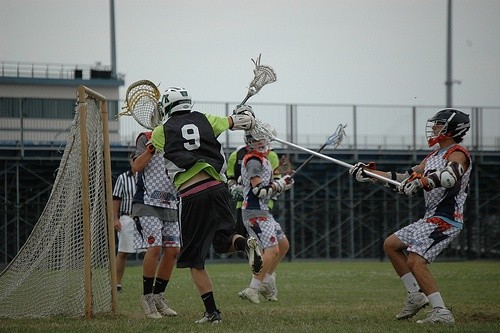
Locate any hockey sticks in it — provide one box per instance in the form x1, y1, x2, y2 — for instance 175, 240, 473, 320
288, 122, 347, 180
125, 79, 164, 131
228, 65, 278, 116
244, 114, 403, 189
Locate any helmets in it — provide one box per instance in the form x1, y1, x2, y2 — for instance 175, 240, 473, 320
149, 102, 164, 128
244, 118, 277, 156
425, 107, 471, 147
161, 87, 193, 119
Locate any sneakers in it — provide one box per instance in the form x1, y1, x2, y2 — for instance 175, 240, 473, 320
258, 282, 278, 302
416, 306, 456, 323
139, 292, 163, 319
395, 288, 429, 320
239, 288, 262, 304
153, 292, 178, 316
194, 309, 222, 324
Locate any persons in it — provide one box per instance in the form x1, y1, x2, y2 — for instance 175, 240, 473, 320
226, 122, 281, 296
151, 86, 255, 324
112, 150, 148, 294
133, 101, 180, 319
239, 124, 294, 303
350, 109, 472, 326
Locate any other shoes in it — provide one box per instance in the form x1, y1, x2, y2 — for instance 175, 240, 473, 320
116, 284, 122, 292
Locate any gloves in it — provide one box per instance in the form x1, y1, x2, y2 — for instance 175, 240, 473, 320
232, 105, 255, 118
349, 161, 377, 184
230, 184, 244, 202
272, 175, 294, 192
399, 171, 428, 197
